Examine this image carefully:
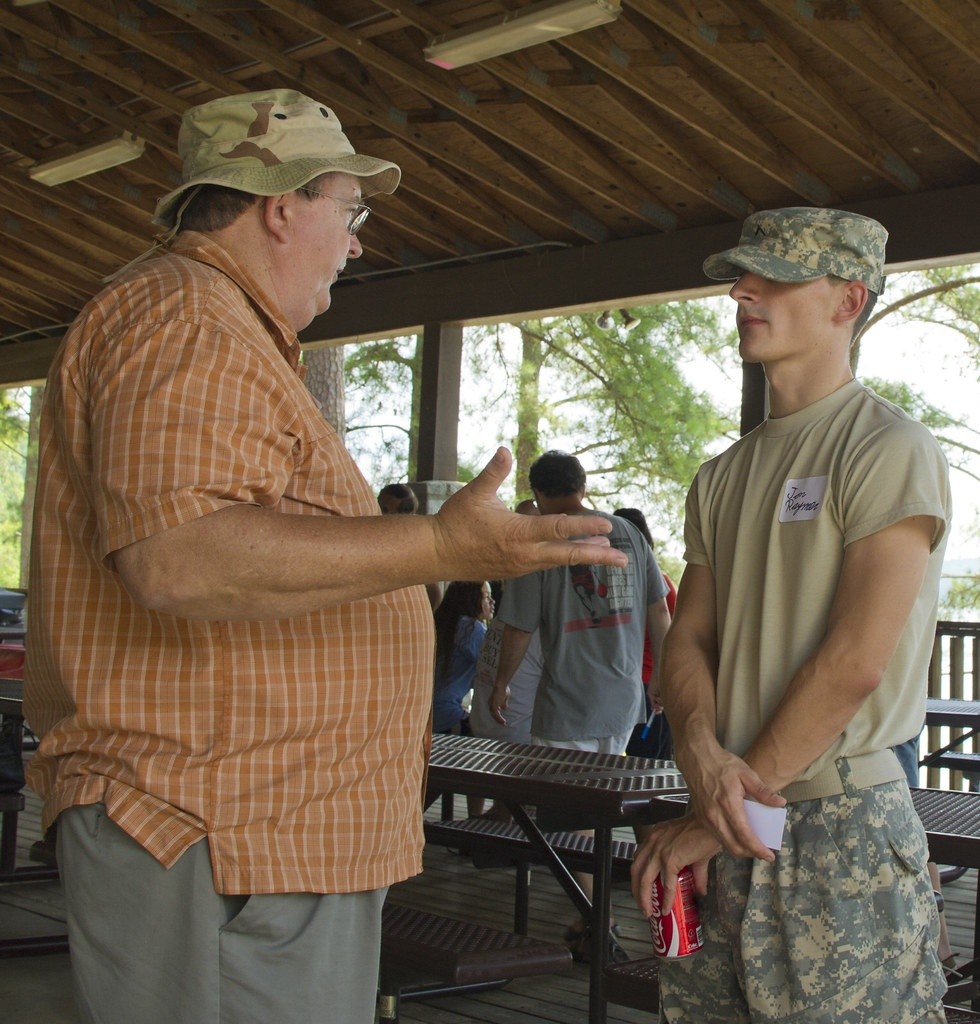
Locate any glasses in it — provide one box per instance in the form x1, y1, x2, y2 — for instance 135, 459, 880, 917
300, 187, 373, 236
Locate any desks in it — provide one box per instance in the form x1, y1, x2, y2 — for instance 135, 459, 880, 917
0, 676, 25, 877
430, 697, 980, 1024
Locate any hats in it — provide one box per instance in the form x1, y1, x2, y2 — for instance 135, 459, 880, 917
155, 88, 402, 230
702, 206, 889, 296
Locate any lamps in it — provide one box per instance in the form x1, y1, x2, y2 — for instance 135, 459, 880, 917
422, 0, 624, 71
28, 130, 145, 187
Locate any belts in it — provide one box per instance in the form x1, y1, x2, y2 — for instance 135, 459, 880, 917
762, 748, 907, 803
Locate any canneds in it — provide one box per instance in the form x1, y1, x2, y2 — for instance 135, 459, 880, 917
648, 865, 704, 960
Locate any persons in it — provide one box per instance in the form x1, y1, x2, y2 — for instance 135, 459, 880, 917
377, 451, 675, 961
628, 205, 958, 1024
22, 87, 630, 1024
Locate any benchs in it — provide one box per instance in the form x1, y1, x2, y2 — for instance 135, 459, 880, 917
607, 956, 980, 1024
923, 751, 980, 792
426, 820, 636, 935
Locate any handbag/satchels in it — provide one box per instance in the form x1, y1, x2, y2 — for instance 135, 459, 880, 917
625, 722, 666, 758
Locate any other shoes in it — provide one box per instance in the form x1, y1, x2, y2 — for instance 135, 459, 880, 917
568, 937, 593, 964
940, 953, 961, 985
563, 917, 621, 943
